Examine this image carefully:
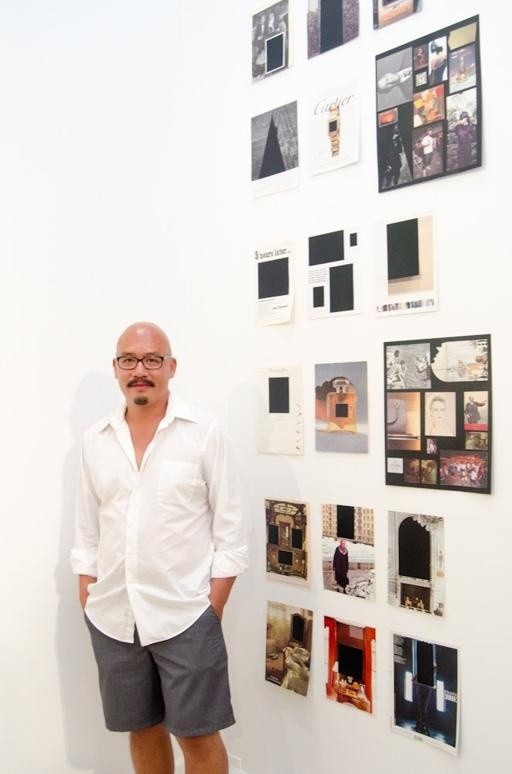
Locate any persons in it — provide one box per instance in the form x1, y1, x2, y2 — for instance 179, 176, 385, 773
465, 395, 486, 424
68, 320, 252, 774
387, 350, 401, 372
333, 539, 350, 594
454, 111, 475, 167
417, 126, 437, 179
414, 354, 428, 378
429, 395, 452, 436
388, 361, 406, 387
377, 49, 412, 102
439, 458, 487, 484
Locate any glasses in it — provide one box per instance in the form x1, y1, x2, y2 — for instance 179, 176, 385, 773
117, 354, 168, 370
